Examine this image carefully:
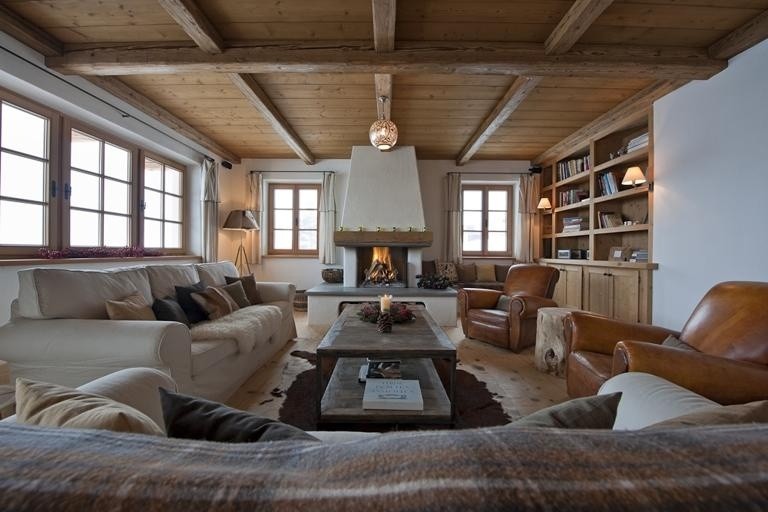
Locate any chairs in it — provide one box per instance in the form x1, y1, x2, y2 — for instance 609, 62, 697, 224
458, 262, 561, 353
561, 279, 768, 405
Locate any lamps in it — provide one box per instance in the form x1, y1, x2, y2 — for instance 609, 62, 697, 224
622, 166, 653, 192
368, 96, 399, 151
222, 209, 261, 278
537, 197, 553, 214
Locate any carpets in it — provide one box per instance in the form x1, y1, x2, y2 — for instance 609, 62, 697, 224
278, 368, 511, 433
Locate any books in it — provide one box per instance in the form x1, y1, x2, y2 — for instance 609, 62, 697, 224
555, 133, 649, 262
364, 379, 424, 411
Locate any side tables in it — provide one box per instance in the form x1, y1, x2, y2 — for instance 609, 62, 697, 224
534, 306, 609, 379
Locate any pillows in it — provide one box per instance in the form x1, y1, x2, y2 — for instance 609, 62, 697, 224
103, 272, 265, 330
501, 391, 768, 433
422, 261, 510, 282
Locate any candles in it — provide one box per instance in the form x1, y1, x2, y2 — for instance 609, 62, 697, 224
380, 294, 390, 313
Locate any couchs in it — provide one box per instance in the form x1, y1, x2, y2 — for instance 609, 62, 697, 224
1, 260, 298, 403
2, 363, 768, 512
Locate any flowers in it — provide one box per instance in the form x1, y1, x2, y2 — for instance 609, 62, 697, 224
415, 274, 453, 290
356, 301, 417, 324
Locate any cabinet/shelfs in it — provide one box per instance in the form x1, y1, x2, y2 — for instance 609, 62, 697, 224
533, 103, 658, 327
